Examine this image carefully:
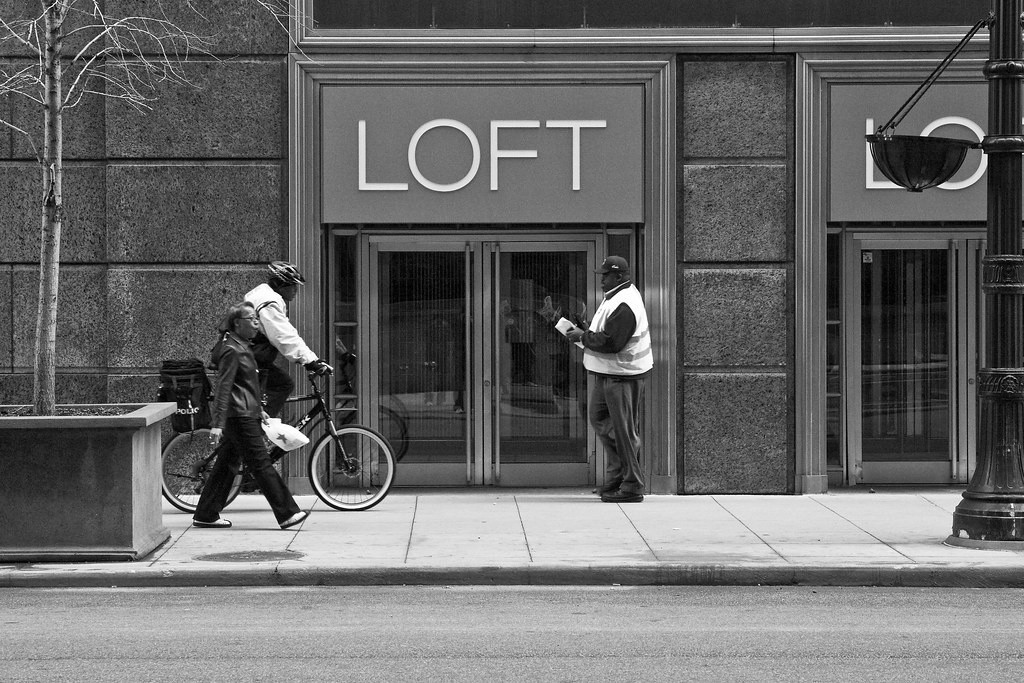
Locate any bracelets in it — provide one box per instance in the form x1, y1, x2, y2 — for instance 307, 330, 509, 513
579, 335, 583, 342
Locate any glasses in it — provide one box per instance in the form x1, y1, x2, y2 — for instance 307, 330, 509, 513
239, 316, 260, 322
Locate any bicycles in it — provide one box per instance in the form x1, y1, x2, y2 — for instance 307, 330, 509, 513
333, 348, 412, 464
158, 365, 398, 512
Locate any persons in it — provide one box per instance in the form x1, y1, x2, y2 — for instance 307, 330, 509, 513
336, 333, 357, 425
453, 306, 473, 414
566, 255, 655, 502
192, 302, 311, 530
219, 260, 335, 492
422, 309, 454, 407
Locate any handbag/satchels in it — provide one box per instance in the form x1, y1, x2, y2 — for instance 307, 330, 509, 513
259, 410, 310, 451
155, 357, 214, 433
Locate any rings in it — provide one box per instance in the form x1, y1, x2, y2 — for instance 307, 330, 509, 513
209, 437, 212, 440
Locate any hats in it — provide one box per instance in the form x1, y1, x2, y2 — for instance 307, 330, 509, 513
593, 255, 629, 273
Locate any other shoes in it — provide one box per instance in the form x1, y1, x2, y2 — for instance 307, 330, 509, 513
592, 474, 624, 493
280, 511, 307, 529
601, 488, 643, 502
425, 401, 434, 407
193, 517, 232, 528
455, 407, 465, 414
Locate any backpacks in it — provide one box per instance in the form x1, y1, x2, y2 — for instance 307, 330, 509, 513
208, 301, 279, 364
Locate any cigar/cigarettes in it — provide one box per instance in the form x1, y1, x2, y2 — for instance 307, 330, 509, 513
210, 442, 219, 444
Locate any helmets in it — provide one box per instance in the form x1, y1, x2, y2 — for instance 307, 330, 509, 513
267, 260, 306, 292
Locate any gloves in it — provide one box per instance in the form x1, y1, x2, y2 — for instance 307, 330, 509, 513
306, 358, 334, 378
339, 352, 357, 363
